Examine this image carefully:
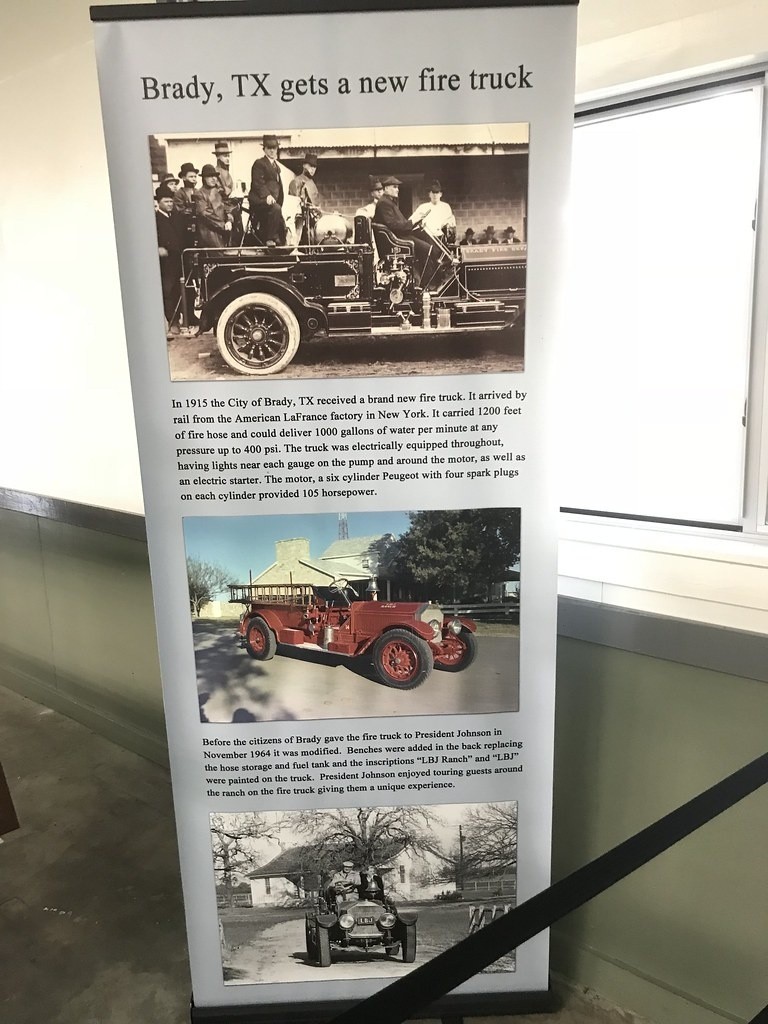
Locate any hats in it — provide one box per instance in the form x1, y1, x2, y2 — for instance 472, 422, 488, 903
504, 226, 516, 233
426, 179, 444, 192
302, 153, 321, 167
259, 135, 281, 148
178, 162, 199, 179
342, 860, 354, 867
212, 142, 233, 154
482, 225, 495, 234
368, 173, 404, 193
464, 228, 475, 234
197, 163, 220, 177
154, 173, 180, 200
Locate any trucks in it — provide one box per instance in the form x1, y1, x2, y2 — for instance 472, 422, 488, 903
227, 576, 477, 690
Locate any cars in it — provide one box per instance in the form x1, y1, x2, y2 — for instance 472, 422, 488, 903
180, 216, 527, 376
306, 870, 419, 967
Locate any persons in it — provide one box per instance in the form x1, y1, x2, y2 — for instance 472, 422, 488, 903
324, 861, 384, 902
155, 135, 522, 341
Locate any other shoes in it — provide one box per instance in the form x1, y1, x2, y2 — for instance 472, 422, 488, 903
266, 239, 276, 247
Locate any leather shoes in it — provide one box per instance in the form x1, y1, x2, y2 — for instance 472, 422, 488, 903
170, 320, 181, 335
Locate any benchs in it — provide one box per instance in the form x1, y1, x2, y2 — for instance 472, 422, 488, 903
374, 223, 413, 259
313, 585, 361, 610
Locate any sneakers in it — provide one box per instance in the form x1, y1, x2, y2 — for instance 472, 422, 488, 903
188, 307, 201, 326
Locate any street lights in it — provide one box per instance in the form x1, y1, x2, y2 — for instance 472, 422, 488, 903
459, 825, 466, 890
